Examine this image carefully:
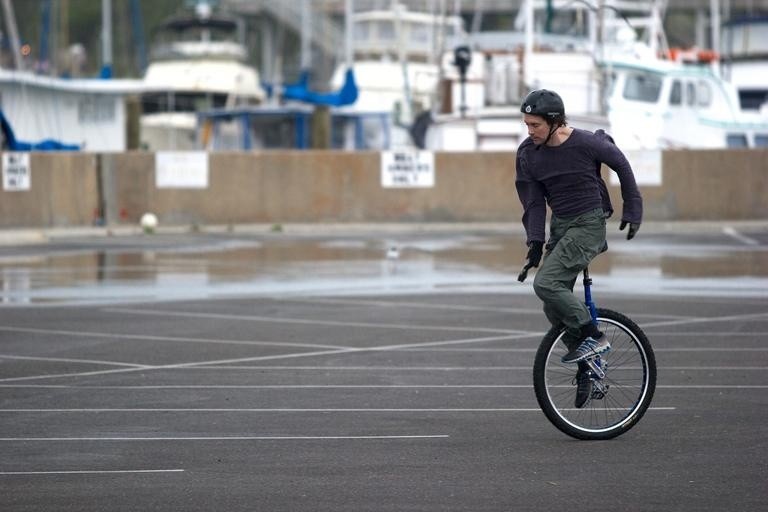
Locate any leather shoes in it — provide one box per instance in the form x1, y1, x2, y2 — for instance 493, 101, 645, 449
618, 222, 641, 241
517, 242, 544, 282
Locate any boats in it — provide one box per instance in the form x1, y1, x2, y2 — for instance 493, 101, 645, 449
0, 1, 768, 153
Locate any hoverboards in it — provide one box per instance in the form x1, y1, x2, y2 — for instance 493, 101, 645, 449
532, 238, 657, 441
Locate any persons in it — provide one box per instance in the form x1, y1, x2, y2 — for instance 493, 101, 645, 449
514, 89, 644, 408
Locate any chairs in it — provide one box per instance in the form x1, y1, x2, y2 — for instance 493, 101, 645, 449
561, 332, 611, 364
573, 370, 593, 409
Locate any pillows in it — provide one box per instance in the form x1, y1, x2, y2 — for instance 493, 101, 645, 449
520, 88, 566, 122
